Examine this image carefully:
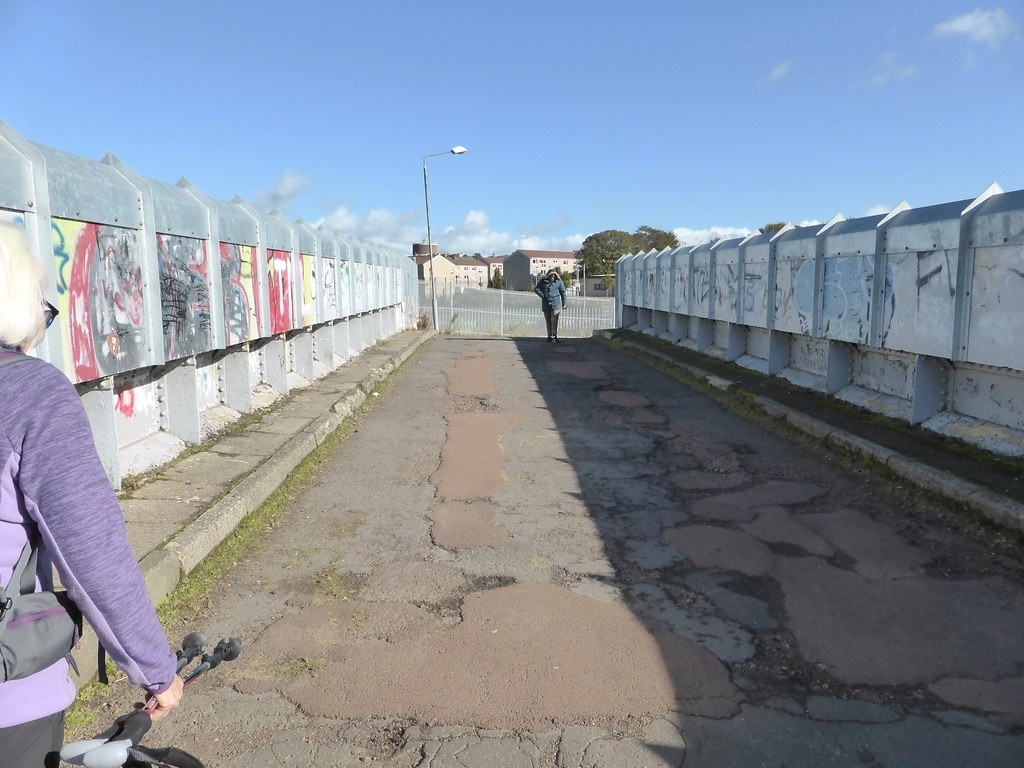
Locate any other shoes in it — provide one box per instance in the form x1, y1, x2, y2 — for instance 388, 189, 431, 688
547, 336, 552, 343
552, 337, 557, 343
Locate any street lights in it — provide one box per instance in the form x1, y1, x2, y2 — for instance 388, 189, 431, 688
423, 146, 467, 329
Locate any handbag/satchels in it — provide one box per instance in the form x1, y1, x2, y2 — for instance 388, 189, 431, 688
0, 588, 83, 682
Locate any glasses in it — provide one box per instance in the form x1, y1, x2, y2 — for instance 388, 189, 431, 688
42, 298, 59, 329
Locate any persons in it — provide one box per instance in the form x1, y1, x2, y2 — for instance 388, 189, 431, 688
0, 221, 184, 768
535, 269, 567, 344
